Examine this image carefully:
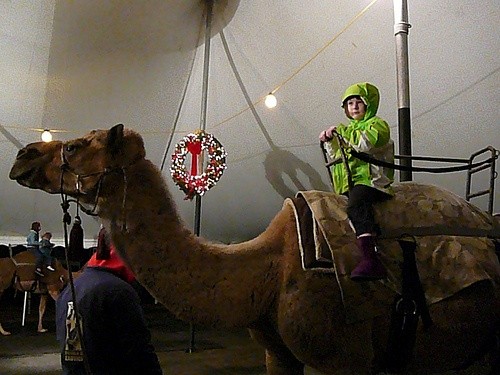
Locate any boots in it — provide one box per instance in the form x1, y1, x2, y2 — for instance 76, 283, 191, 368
350, 235, 388, 282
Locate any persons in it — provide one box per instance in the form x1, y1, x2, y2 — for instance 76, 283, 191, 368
39, 232, 55, 272
57, 228, 162, 375
319, 82, 394, 280
27, 222, 45, 276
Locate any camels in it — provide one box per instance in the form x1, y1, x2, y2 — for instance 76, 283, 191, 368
0, 242, 89, 334
8, 122, 500, 375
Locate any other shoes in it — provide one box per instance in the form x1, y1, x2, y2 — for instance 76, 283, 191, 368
35, 269, 44, 277
47, 266, 56, 272
